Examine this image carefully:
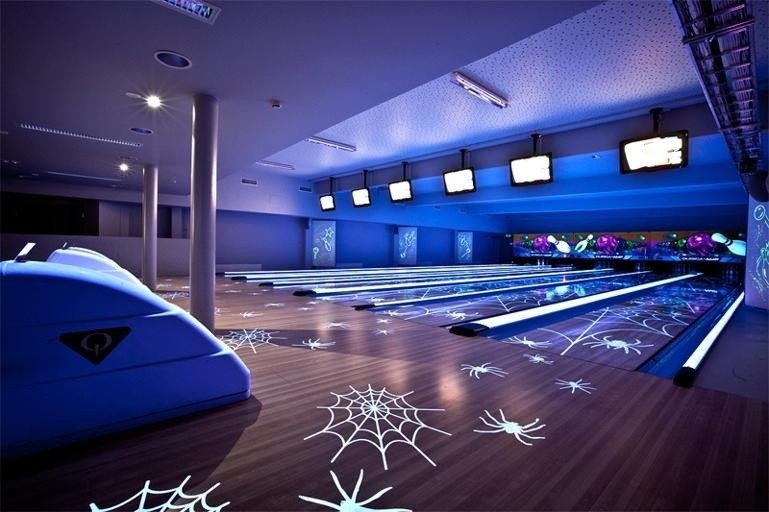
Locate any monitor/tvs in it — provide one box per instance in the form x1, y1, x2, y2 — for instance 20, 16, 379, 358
351, 187, 371, 207
509, 152, 553, 186
319, 194, 336, 212
388, 179, 413, 203
443, 166, 476, 196
620, 129, 688, 174
